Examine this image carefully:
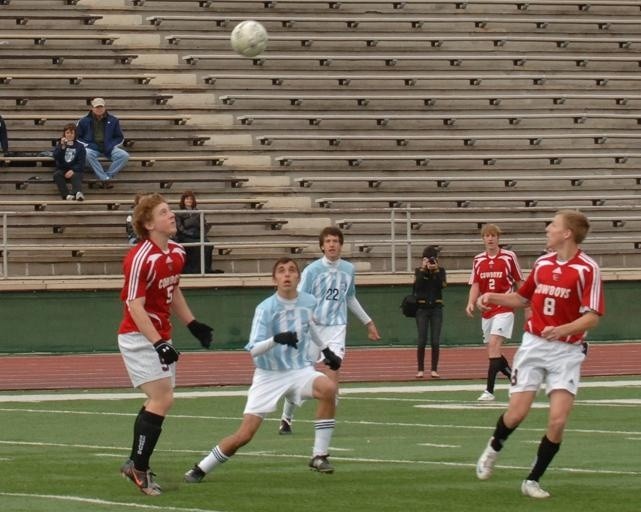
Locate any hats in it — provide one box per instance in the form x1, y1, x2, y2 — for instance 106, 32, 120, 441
89, 95, 104, 107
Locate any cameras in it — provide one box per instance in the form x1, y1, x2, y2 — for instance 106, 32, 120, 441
428, 257, 437, 264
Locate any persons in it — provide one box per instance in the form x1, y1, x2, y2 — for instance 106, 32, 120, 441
53, 124, 87, 200
477, 209, 605, 497
402, 246, 450, 378
118, 191, 215, 495
126, 192, 146, 245
465, 224, 530, 401
278, 226, 380, 434
75, 97, 130, 189
172, 190, 225, 272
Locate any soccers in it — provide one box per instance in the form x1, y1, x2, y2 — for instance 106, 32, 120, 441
231, 21, 267, 56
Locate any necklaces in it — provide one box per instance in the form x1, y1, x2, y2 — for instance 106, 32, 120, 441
185, 258, 343, 482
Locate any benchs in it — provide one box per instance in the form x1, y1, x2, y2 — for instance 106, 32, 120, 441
1, 2, 639, 276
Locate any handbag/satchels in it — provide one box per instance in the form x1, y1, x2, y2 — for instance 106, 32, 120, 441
399, 294, 418, 319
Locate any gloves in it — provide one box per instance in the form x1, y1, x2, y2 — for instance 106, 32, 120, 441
273, 329, 300, 351
152, 339, 179, 372
320, 345, 342, 371
184, 315, 215, 348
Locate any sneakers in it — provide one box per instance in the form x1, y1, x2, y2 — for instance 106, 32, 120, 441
518, 478, 550, 499
182, 465, 207, 485
476, 435, 501, 480
277, 416, 292, 435
413, 369, 439, 380
307, 454, 335, 474
61, 182, 116, 202
475, 390, 496, 403
120, 457, 164, 490
122, 462, 159, 497
